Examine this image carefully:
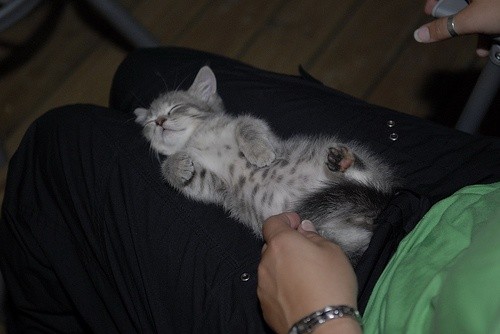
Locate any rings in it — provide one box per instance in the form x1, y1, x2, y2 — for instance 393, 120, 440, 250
448, 15, 458, 38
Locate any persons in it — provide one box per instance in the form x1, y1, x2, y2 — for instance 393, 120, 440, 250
0, 0, 500, 333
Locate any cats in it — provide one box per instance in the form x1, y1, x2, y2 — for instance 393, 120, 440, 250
133, 66, 397, 271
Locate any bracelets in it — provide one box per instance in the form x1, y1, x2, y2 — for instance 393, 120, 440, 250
287, 303, 362, 333
